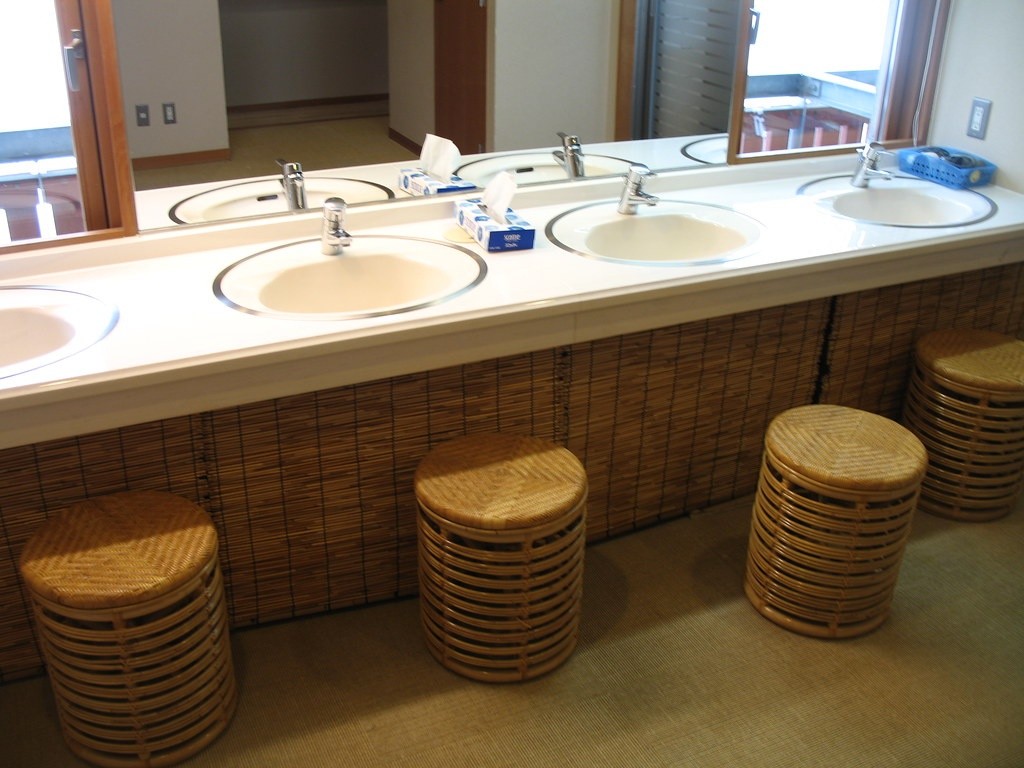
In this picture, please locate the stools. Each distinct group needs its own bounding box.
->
[745,405,927,638]
[413,432,591,683]
[904,326,1023,521]
[19,489,236,768]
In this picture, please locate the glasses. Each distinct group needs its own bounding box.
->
[921,147,977,169]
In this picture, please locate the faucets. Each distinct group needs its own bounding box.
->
[274,157,308,210]
[853,141,894,188]
[619,163,660,215]
[319,196,352,257]
[552,131,585,177]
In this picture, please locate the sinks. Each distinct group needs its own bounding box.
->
[257,253,450,313]
[203,190,347,222]
[829,188,974,225]
[0,305,76,368]
[479,162,614,183]
[584,213,748,259]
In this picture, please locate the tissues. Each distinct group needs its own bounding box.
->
[454,170,536,251]
[400,133,476,196]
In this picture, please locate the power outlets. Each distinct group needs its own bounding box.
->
[967,98,992,139]
[136,106,151,126]
[162,103,176,123]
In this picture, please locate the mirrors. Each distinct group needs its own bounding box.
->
[0,0,949,252]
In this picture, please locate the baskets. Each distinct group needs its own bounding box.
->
[898,144,998,191]
